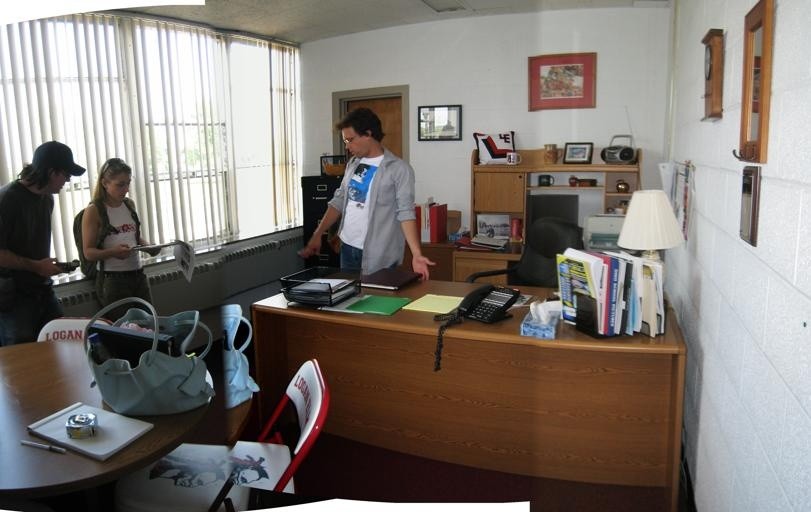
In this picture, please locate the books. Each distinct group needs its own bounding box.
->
[361,267,423,290]
[28,401,155,463]
[555,247,666,338]
[413,196,448,243]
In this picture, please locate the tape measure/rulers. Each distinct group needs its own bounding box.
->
[66,413,98,439]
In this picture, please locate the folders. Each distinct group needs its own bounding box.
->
[86,323,177,370]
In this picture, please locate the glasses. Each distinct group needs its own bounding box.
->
[50,166,72,182]
[102,158,125,175]
[342,128,370,145]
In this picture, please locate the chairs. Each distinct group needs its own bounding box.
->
[226,354,333,511]
[462,216,584,287]
[34,315,113,345]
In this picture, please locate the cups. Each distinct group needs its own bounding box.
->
[511,217,523,239]
[507,152,522,166]
[538,174,555,186]
[544,143,563,165]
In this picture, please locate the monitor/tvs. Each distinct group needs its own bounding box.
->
[527,193,579,224]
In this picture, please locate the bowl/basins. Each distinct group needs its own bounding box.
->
[615,208,624,214]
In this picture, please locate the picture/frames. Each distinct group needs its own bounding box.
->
[562,141,593,164]
[737,166,764,249]
[525,50,599,115]
[474,212,513,237]
[416,103,465,141]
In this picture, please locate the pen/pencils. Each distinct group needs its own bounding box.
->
[20,438,67,454]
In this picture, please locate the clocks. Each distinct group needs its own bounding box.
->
[699,26,728,124]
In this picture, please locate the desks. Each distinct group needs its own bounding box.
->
[1,337,214,511]
[219,388,254,440]
[247,285,691,511]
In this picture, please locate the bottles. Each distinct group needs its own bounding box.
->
[569,175,577,187]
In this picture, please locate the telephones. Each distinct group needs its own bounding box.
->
[457,284,520,325]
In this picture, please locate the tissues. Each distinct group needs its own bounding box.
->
[520,298,563,342]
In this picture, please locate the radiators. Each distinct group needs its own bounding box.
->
[50,257,217,331]
[218,233,304,301]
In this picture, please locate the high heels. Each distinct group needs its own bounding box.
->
[78,295,217,418]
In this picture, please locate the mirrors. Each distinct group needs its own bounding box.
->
[734,0,776,166]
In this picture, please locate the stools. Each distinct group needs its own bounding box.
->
[116,440,235,512]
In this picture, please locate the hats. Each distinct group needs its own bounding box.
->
[33,140,87,177]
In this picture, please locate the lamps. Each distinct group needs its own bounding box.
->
[615,188,687,289]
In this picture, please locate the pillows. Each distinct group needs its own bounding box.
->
[471,129,517,167]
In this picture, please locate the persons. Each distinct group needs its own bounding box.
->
[77,157,161,321]
[486,225,495,238]
[0,140,87,347]
[296,106,437,283]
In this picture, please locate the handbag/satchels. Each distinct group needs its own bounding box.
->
[215,302,263,411]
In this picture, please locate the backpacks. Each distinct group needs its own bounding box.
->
[72,199,141,279]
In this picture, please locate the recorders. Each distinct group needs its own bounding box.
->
[601,135,636,164]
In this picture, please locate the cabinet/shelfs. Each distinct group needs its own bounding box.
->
[451,243,523,283]
[398,208,461,283]
[466,147,644,243]
[298,173,344,269]
